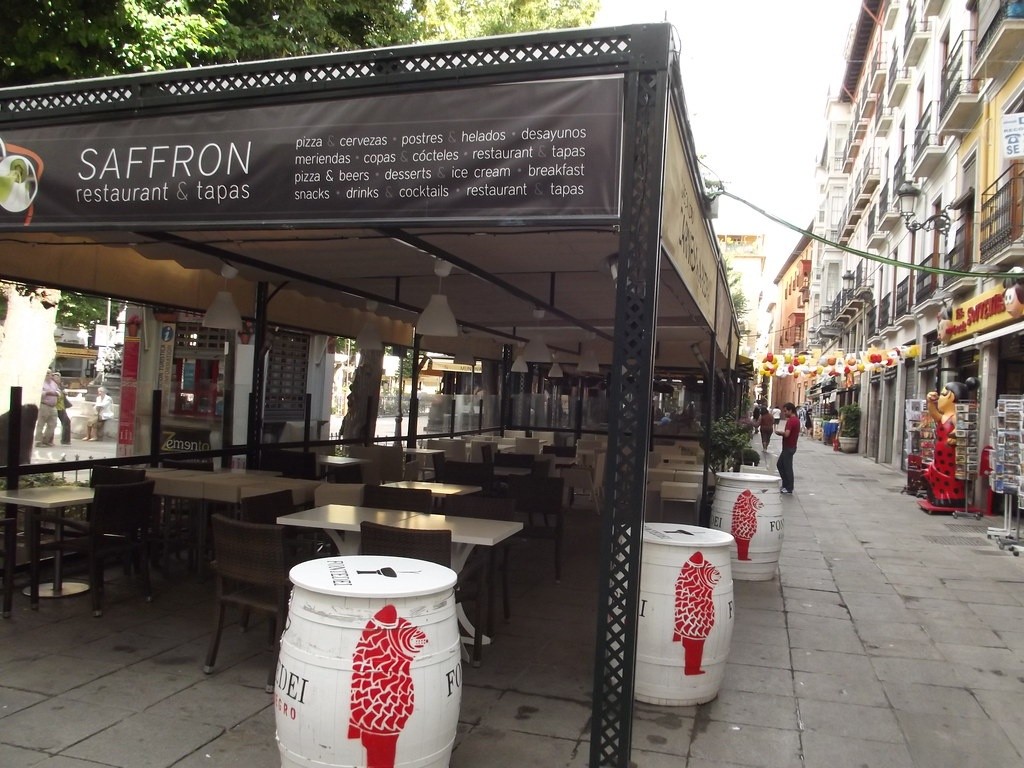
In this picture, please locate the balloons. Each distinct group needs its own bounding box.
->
[757,344,922,380]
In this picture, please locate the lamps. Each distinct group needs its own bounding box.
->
[842,271,875,304]
[896,180,952,239]
[520,307,551,363]
[454,333,474,365]
[576,330,599,372]
[415,258,460,339]
[201,290,243,332]
[822,307,843,332]
[547,353,563,378]
[509,341,528,373]
[355,310,384,352]
[809,327,830,347]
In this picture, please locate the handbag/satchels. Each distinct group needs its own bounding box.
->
[64,396,72,408]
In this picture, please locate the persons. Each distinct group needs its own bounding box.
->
[34,368,62,447]
[935,306,953,343]
[754,407,774,453]
[753,400,765,433]
[774,402,802,494]
[81,387,113,442]
[927,377,982,507]
[796,404,814,437]
[1002,276,1024,318]
[52,373,72,446]
[770,405,782,429]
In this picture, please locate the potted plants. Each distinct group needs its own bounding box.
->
[839,402,861,453]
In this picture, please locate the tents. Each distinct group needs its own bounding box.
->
[0,23,752,768]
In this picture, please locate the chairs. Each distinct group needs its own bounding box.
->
[0,429,726,698]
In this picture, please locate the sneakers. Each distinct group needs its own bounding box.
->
[781,487,788,493]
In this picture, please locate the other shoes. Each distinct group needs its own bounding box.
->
[35,442,54,447]
[82,436,103,441]
[61,443,73,447]
